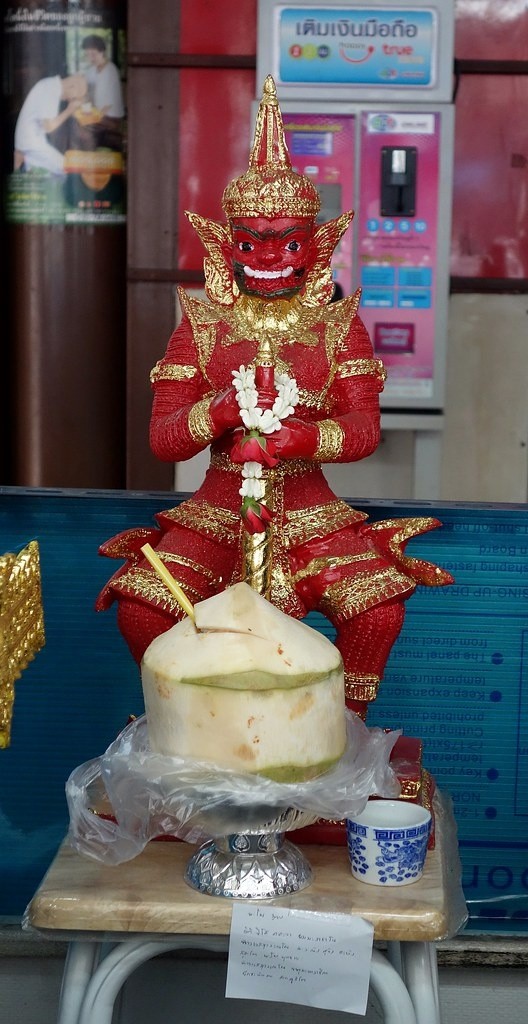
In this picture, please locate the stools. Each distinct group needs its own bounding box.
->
[27,786,449,1024]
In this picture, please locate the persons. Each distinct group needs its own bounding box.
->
[13,34,126,177]
[93,73,457,730]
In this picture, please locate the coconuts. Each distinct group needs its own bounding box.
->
[141,583,346,780]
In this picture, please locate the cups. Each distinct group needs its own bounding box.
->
[347,799,432,888]
